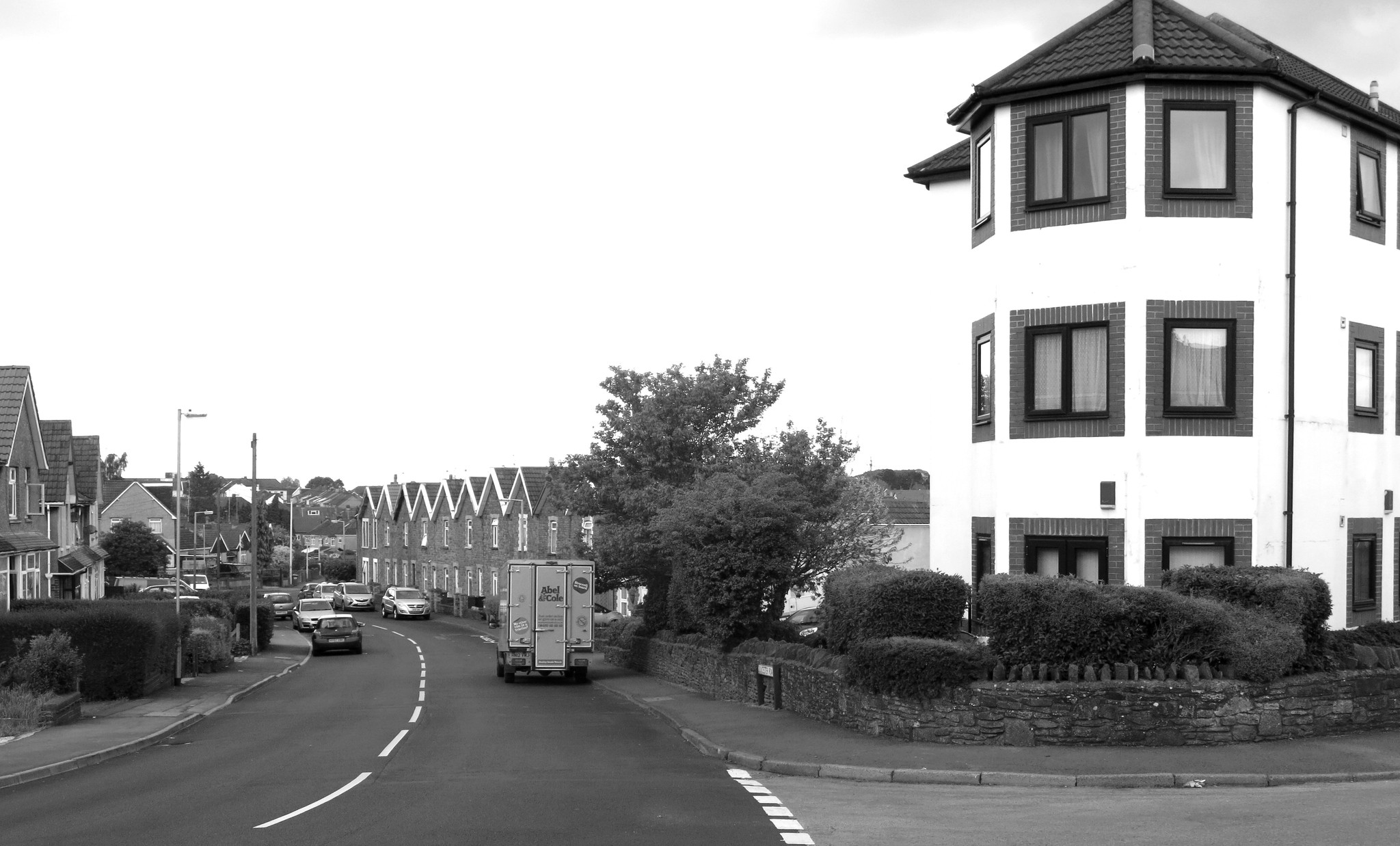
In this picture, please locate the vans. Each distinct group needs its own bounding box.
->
[183,574,212,590]
[115,577,197,591]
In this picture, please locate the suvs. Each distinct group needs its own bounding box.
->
[381,586,430,620]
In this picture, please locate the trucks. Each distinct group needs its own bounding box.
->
[488,559,596,683]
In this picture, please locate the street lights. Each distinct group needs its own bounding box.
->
[499,498,524,559]
[193,511,213,589]
[331,519,345,560]
[172,409,207,683]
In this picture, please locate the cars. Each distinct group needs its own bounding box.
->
[292,598,335,632]
[297,583,319,600]
[312,584,338,602]
[310,614,365,656]
[139,584,187,594]
[594,603,623,625]
[332,582,375,612]
[262,593,295,620]
